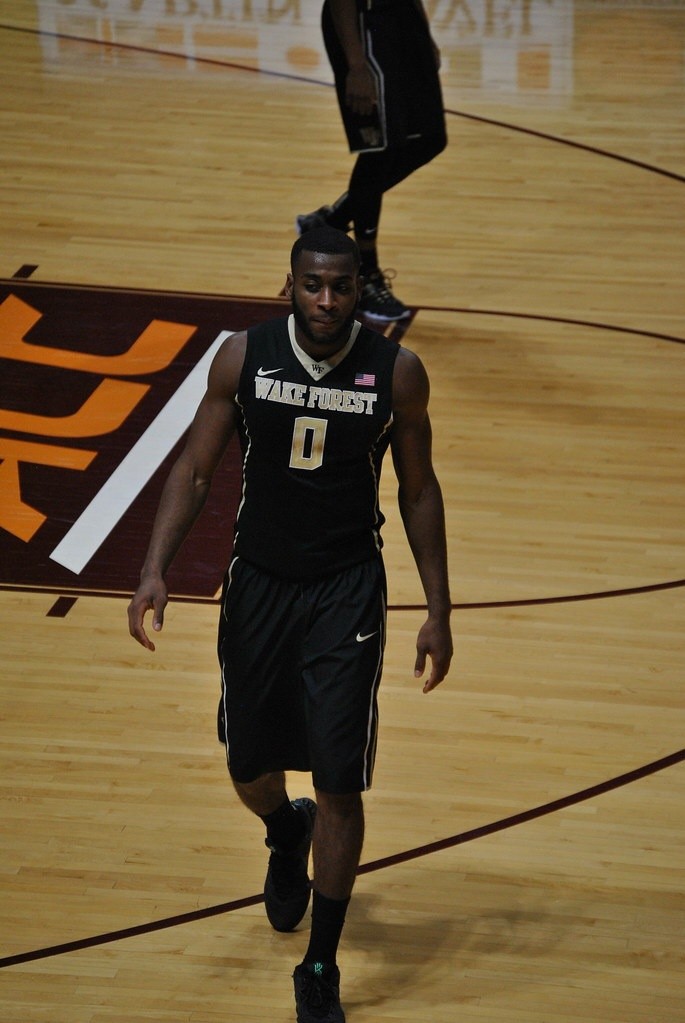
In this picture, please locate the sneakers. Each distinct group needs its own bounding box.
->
[358,268,411,321]
[296,203,351,237]
[291,963,345,1023]
[264,797,317,932]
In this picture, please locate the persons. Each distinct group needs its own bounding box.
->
[297,0,448,321]
[128,228,453,1023]
